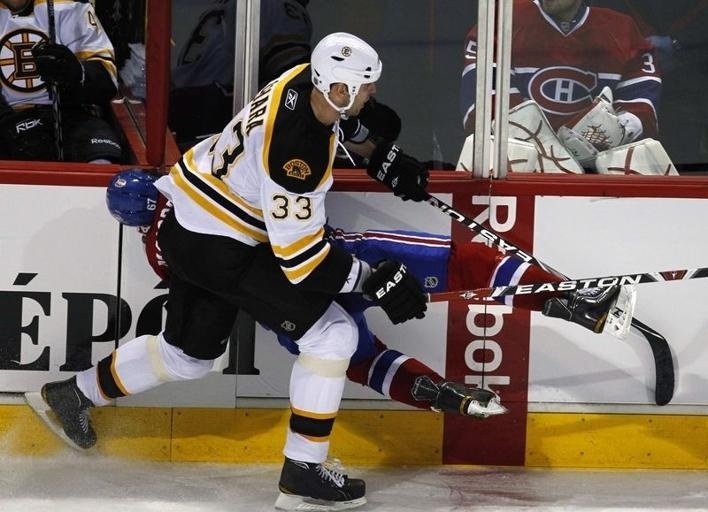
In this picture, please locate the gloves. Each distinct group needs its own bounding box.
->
[32,43,83,85]
[362,257,428,323]
[367,142,429,201]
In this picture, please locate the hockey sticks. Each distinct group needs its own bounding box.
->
[422,189,675,406]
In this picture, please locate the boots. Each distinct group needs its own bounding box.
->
[41,376,97,449]
[279,457,365,500]
[542,284,621,333]
[411,375,495,416]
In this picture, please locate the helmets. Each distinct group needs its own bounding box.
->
[106,169,158,225]
[311,32,383,97]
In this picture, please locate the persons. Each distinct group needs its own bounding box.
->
[453,0,680,176]
[39,32,430,503]
[0,1,131,165]
[162,0,317,149]
[104,163,622,418]
